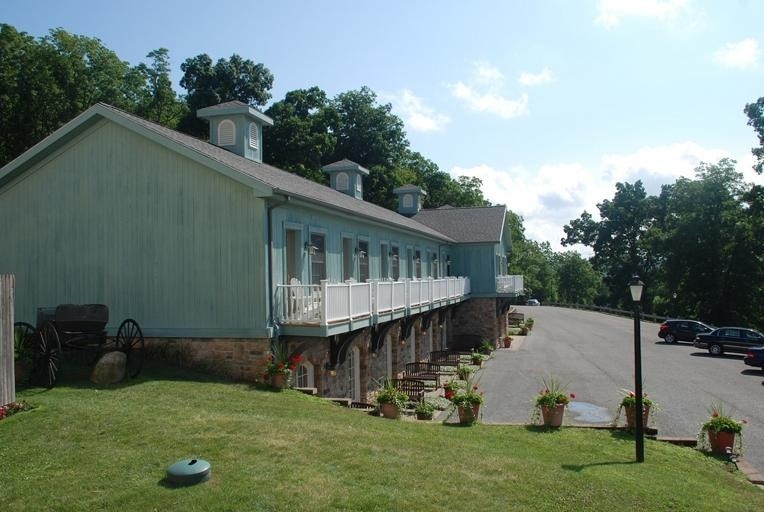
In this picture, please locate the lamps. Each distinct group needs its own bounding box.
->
[304,242,318,256]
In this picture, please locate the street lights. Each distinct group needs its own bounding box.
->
[627,273,646,464]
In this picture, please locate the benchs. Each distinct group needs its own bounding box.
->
[392,340,475,408]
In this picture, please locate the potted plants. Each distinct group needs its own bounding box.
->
[470,337,496,365]
[503,336,513,348]
[265,341,304,380]
[371,376,434,420]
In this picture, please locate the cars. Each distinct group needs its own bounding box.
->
[657,319,714,345]
[526,299,541,306]
[693,326,764,357]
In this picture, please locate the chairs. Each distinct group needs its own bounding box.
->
[290,277,314,320]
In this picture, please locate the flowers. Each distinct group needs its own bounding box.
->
[696,399,748,451]
[616,376,657,418]
[444,370,488,420]
[530,371,575,420]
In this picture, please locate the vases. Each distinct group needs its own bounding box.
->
[625,407,649,426]
[541,404,564,427]
[457,402,479,424]
[708,430,735,453]
[270,371,289,389]
